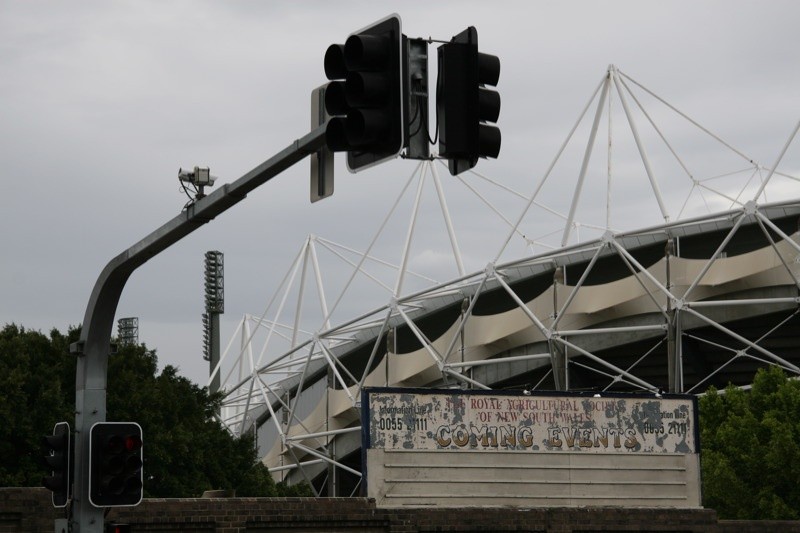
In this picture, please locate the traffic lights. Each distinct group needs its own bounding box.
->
[90,422,144,508]
[323,13,403,174]
[438,26,501,175]
[40,422,69,508]
